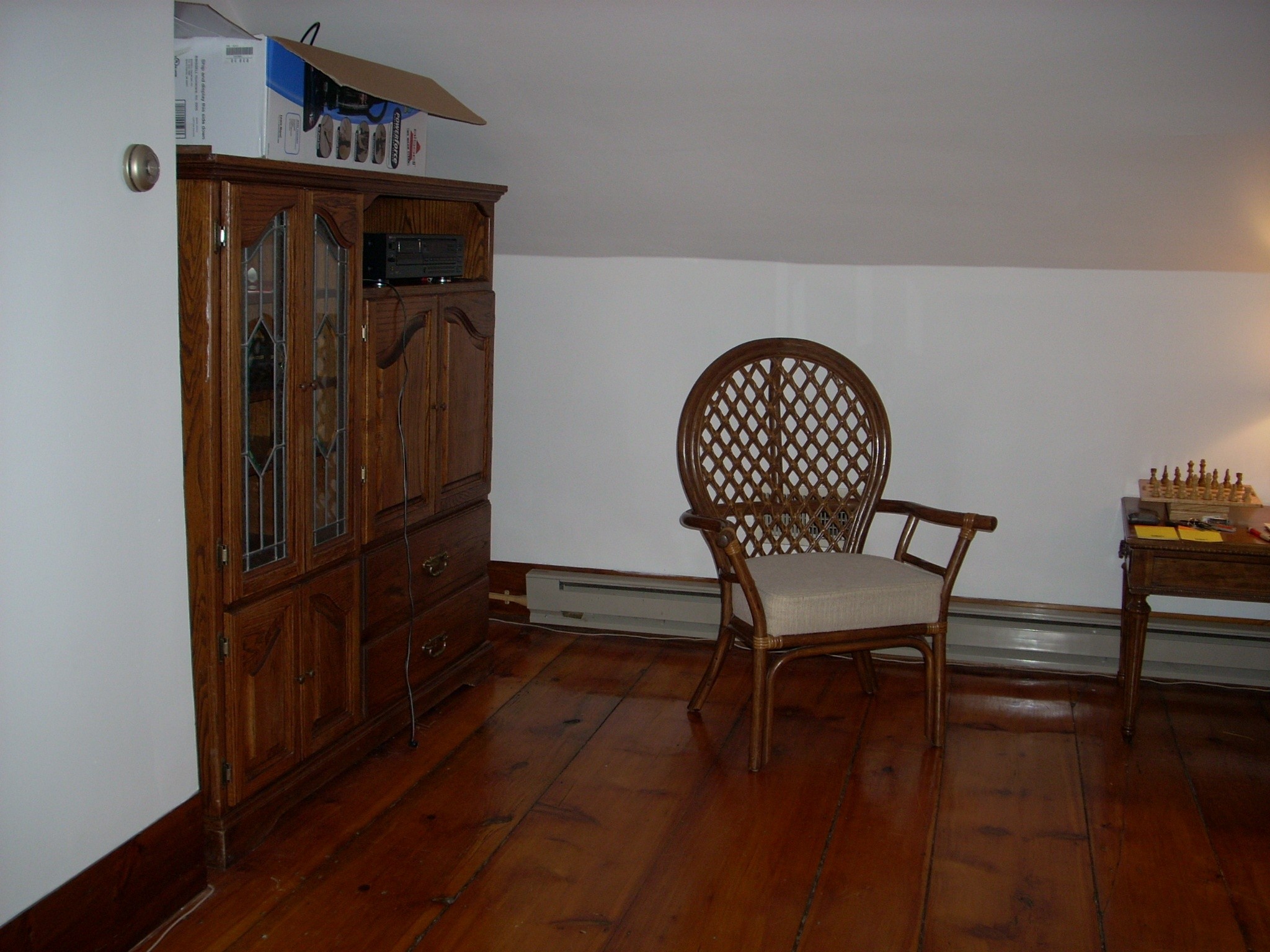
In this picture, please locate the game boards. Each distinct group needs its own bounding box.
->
[1138,479,1263,506]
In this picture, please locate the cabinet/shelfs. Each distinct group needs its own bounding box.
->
[177,153,509,873]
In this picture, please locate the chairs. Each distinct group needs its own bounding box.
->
[675,337,999,774]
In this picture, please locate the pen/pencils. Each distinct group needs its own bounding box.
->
[1246,527,1270,542]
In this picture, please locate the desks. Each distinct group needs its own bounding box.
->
[1114,497,1270,746]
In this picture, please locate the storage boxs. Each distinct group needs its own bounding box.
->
[173,1,489,179]
